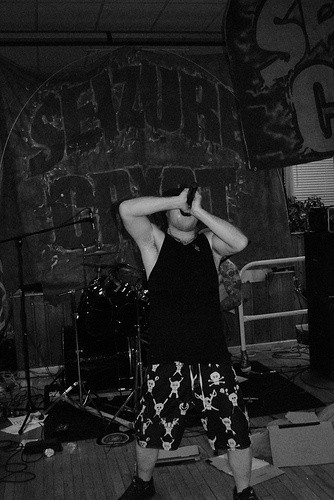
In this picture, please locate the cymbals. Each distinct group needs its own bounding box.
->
[77,250,119,257]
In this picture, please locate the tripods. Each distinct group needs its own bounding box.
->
[0,217,89,436]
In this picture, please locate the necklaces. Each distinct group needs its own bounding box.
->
[168,226,196,245]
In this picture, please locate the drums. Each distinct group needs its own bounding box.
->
[62,326,132,394]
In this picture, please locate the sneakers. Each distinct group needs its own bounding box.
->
[232,486,260,500]
[117,476,156,499]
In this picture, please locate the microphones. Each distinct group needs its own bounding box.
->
[180,182,198,216]
[89,211,95,230]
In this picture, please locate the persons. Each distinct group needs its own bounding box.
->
[112,181,261,500]
[188,224,243,312]
